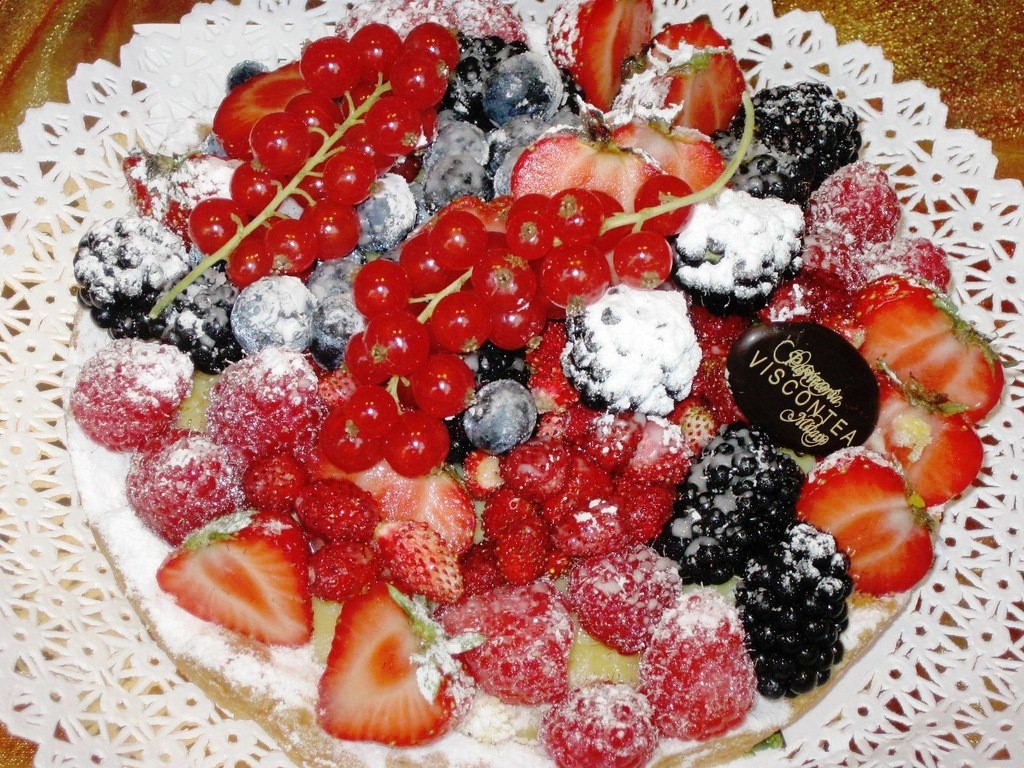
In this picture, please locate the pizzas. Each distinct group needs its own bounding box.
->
[60,0,1005,768]
